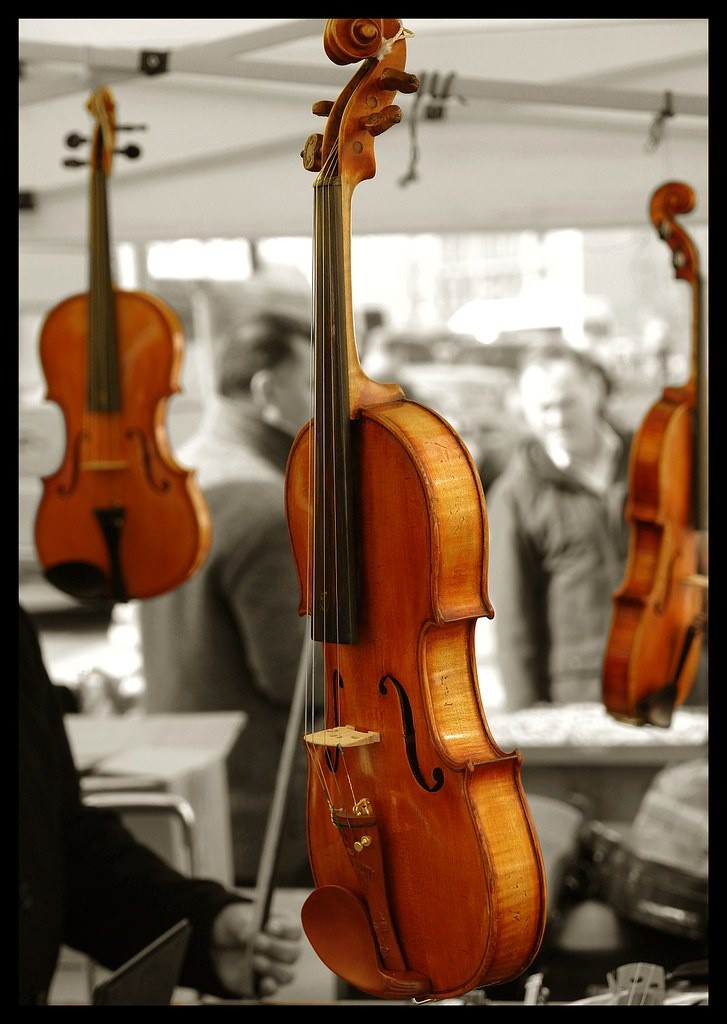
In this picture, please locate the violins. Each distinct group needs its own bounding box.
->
[34,83,214,605]
[284,20,549,1005]
[600,182,708,729]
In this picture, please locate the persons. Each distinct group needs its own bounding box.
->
[15,603,305,1007]
[146,311,642,890]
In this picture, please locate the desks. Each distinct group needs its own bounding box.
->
[64,709,249,889]
[497,704,709,826]
